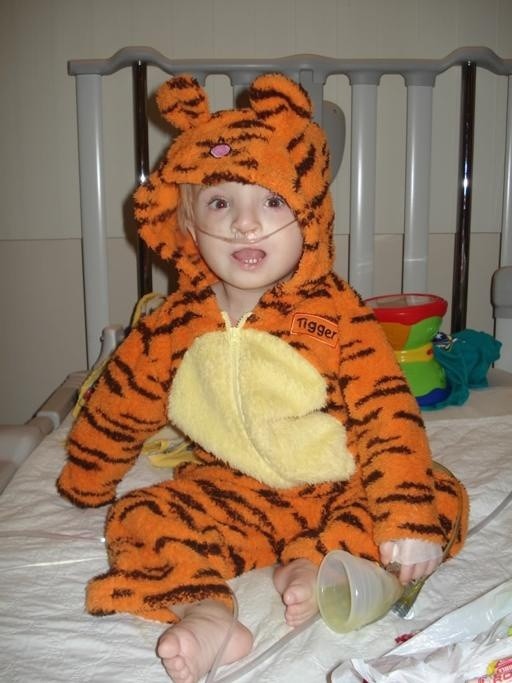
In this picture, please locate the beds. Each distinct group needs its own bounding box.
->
[1,45,512,683]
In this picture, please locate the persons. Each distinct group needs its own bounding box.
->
[54,71,468,683]
